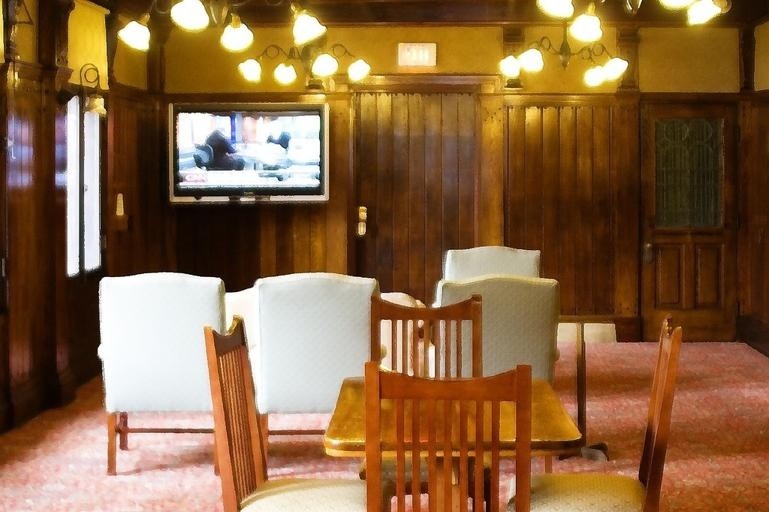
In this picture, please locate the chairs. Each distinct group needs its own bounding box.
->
[357,292,497,512]
[367,357,533,511]
[199,311,368,512]
[504,314,683,511]
[98,240,565,476]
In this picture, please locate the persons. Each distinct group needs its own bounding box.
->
[267,126,292,170]
[195,121,245,171]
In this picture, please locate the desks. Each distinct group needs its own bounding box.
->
[322,370,586,509]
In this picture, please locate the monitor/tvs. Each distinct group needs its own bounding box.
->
[167,103,329,205]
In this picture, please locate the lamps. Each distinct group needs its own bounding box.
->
[495,0,731,92]
[59,62,107,118]
[117,0,372,86]
